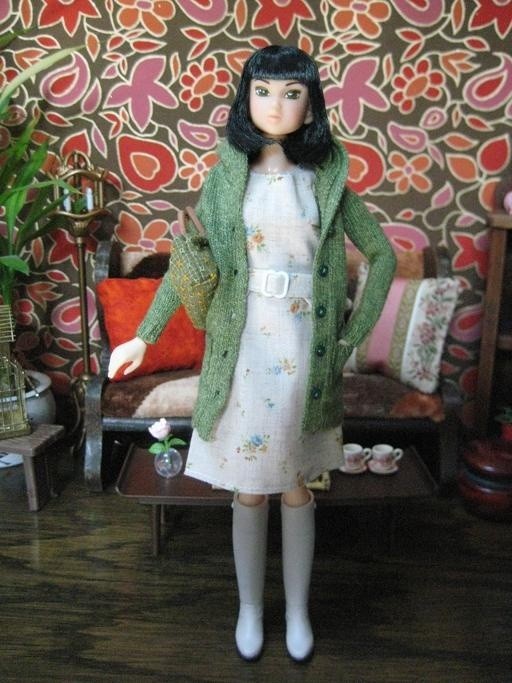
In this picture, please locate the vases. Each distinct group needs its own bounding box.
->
[155,447,182,477]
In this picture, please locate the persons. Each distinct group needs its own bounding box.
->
[108,45,399,661]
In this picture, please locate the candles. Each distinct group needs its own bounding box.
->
[85,187,94,210]
[62,187,70,211]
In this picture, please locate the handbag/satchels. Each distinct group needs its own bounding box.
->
[169,209,220,330]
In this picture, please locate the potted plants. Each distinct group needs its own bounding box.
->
[496,406,512,440]
[0,24,99,440]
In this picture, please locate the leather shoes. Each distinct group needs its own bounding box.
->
[247,268,315,301]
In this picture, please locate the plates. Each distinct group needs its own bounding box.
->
[368,459,398,474]
[338,465,366,473]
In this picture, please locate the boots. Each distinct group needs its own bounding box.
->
[232,492,268,660]
[281,493,315,662]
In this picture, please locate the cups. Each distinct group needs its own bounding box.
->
[341,443,371,469]
[371,443,403,468]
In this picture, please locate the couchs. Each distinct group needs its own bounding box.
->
[83,239,462,491]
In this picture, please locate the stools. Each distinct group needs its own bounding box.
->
[0,423,65,511]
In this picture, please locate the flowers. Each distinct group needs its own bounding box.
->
[147,417,187,468]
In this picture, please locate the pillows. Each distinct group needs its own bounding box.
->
[93,277,204,380]
[346,260,458,393]
